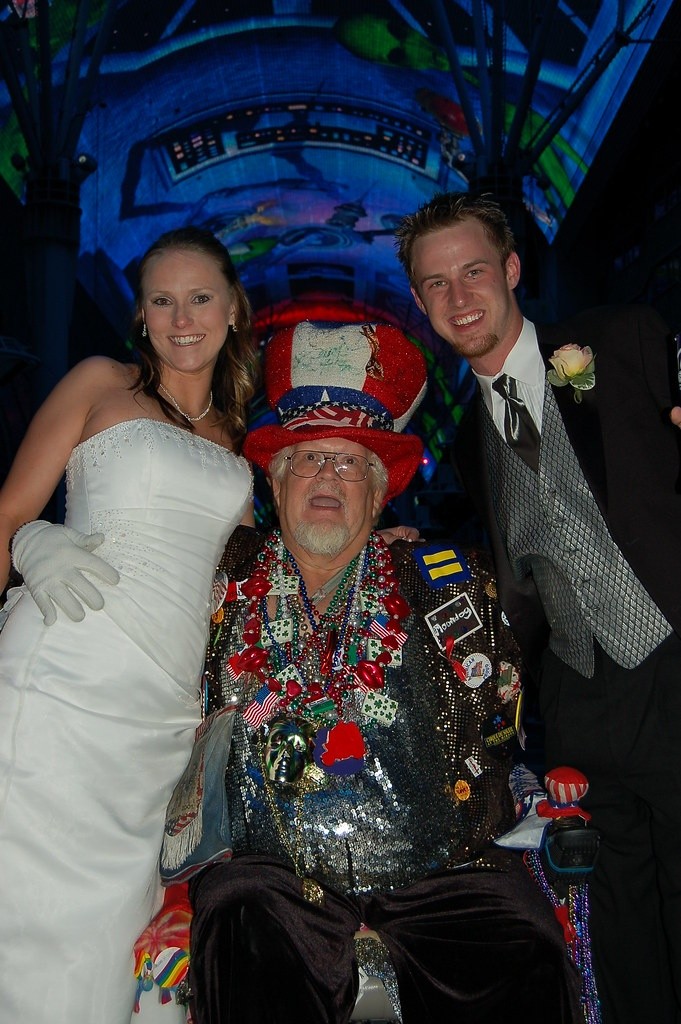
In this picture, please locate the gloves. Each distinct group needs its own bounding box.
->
[10,519,121,626]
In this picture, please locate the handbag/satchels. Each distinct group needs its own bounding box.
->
[158,705,234,887]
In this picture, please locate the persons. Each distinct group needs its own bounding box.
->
[0,227,429,1024]
[188,320,582,1024]
[395,191,681,1024]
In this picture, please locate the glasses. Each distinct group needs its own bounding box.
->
[283,450,375,482]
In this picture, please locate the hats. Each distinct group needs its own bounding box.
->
[241,319,428,508]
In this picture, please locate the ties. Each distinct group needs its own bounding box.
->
[492,373,541,475]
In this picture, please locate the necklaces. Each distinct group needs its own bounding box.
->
[235,524,410,906]
[159,384,213,421]
